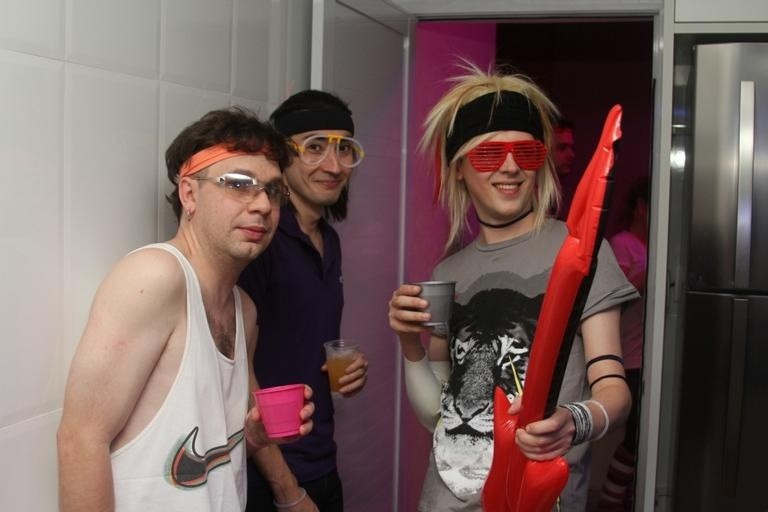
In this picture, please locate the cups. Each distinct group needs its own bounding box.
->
[252,384,306,438]
[325,339,359,395]
[411,280,457,326]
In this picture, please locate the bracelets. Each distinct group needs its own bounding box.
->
[560,395,608,445]
[274,487,307,509]
[588,374,630,391]
[585,354,624,374]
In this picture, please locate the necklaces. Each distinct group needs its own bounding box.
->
[478,206,532,229]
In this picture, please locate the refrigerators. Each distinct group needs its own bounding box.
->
[667,42,763,510]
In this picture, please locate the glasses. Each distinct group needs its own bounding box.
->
[193,172,291,206]
[285,134,366,168]
[463,140,549,172]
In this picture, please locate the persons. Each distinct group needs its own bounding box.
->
[600,180,650,512]
[56,107,315,512]
[551,130,581,221]
[388,53,640,512]
[237,90,370,512]
